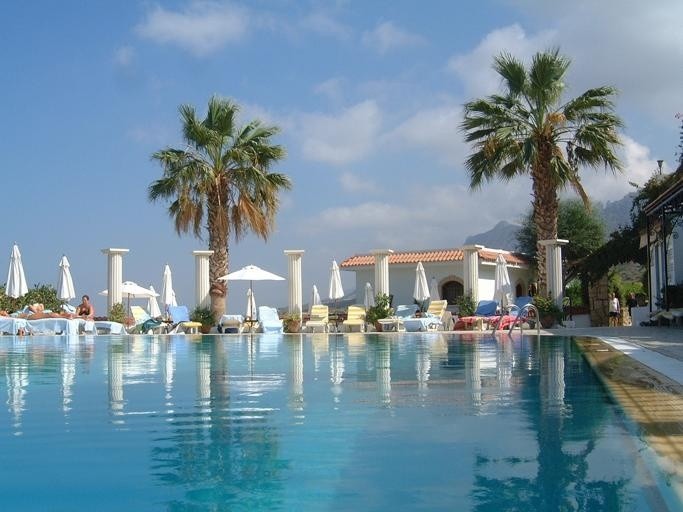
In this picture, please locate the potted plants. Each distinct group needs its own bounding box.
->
[283,313,303,332]
[532,297,557,329]
[193,305,218,332]
[368,294,393,331]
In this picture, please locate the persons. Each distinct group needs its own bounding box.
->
[626,292,637,325]
[413,309,427,318]
[607,293,621,328]
[0,295,95,337]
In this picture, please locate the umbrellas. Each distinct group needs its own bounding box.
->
[308,283,322,316]
[55,254,76,311]
[413,260,430,316]
[492,253,512,318]
[327,256,345,330]
[98,280,160,331]
[430,276,439,301]
[363,282,374,313]
[216,264,287,319]
[161,262,172,321]
[146,283,161,320]
[5,240,29,312]
[161,289,178,312]
[246,288,256,321]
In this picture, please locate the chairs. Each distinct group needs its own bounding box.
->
[129,305,201,335]
[1,303,123,336]
[306,303,365,332]
[257,307,284,332]
[218,314,244,333]
[379,296,536,331]
